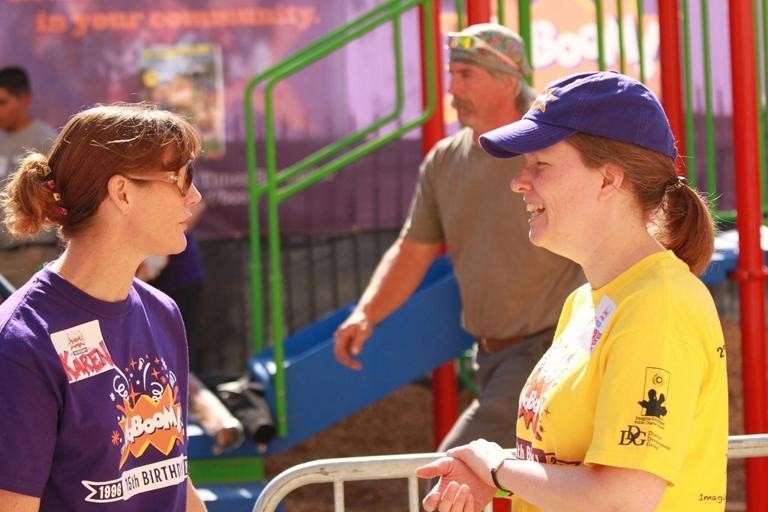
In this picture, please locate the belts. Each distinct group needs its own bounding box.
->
[475,327,548,355]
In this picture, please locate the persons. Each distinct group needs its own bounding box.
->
[0,103,209,512]
[415,70,730,512]
[333,22,590,453]
[0,65,66,291]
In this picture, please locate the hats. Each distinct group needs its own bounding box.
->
[479,70,678,160]
[445,23,530,78]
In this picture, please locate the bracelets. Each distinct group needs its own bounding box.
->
[491,458,515,497]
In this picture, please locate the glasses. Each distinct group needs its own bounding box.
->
[448,35,483,54]
[125,161,194,194]
